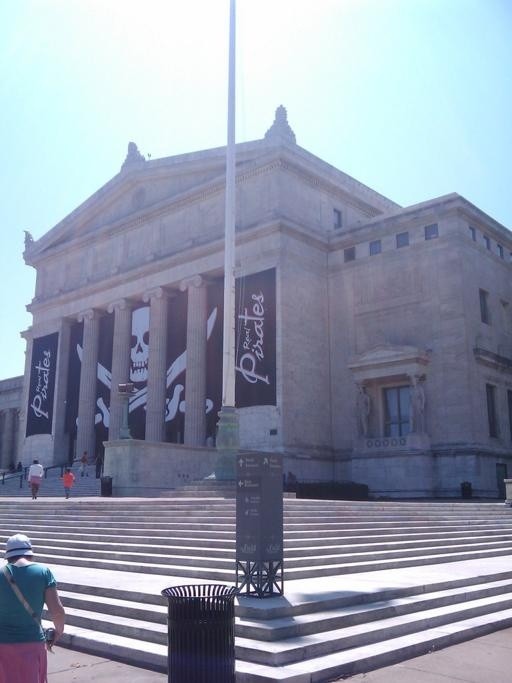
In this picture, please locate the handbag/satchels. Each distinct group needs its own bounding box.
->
[30,476,40,484]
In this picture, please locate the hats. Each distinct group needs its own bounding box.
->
[6,535,33,561]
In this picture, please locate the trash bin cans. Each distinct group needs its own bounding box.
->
[100,476,112,496]
[161,584,240,683]
[461,482,472,499]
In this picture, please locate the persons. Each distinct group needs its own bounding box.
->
[58,467,77,500]
[78,450,90,476]
[28,458,45,499]
[93,449,102,479]
[1,529,68,682]
